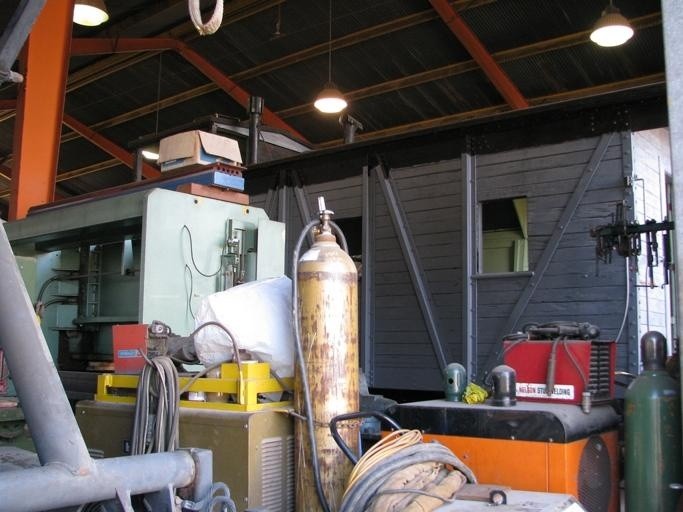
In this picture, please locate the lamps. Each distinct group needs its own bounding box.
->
[588,1,635,46]
[70,1,113,28]
[312,1,346,117]
[141,33,174,162]
[338,109,362,144]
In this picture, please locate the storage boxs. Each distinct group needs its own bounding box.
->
[156,128,242,175]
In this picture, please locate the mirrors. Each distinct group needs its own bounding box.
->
[472,194,530,275]
[325,212,365,279]
